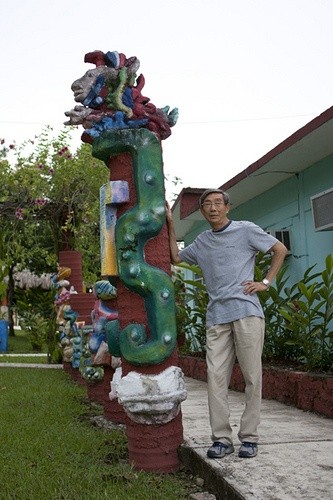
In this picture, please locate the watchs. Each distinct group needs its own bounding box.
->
[262,278,270,287]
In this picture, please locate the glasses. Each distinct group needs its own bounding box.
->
[202,202,223,207]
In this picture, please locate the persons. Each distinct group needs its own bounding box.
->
[165,189,288,458]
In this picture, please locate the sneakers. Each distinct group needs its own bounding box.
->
[239,442,258,458]
[207,443,235,458]
